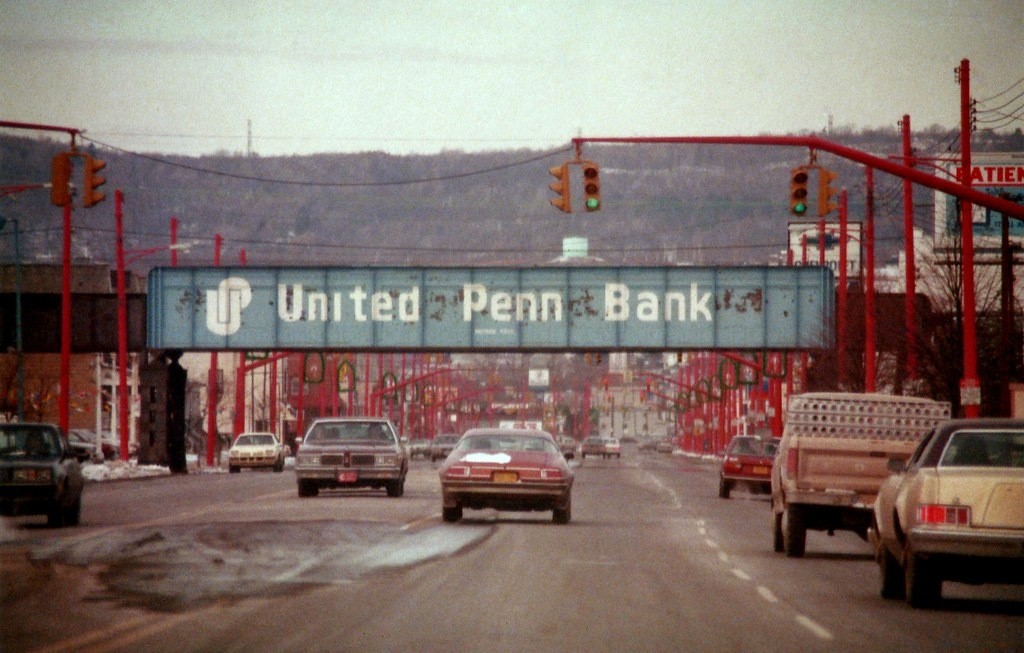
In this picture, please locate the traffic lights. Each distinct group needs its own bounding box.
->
[819,168,837,215]
[549,165,570,214]
[84,156,107,209]
[582,164,601,211]
[790,169,808,216]
[595,352,600,366]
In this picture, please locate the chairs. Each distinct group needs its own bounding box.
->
[952,436,990,467]
[475,439,491,449]
[327,427,340,438]
[23,431,44,453]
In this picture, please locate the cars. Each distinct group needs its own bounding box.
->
[430,434,461,461]
[68,428,132,461]
[604,439,621,458]
[408,439,431,457]
[438,428,576,523]
[228,432,285,473]
[0,422,83,526]
[581,437,607,459]
[871,416,1024,609]
[656,442,673,453]
[719,435,781,498]
[294,418,410,497]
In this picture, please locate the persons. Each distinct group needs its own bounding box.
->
[314,424,327,440]
[367,423,385,439]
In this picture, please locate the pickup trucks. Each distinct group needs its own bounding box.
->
[765,393,922,558]
